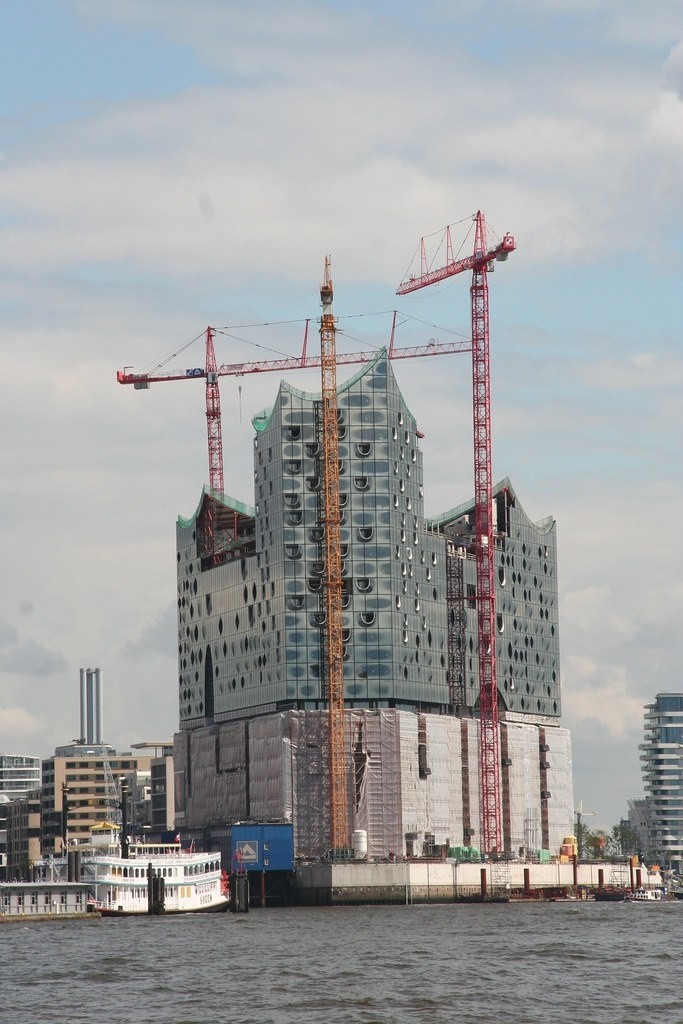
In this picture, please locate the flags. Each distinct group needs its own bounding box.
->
[174,833,180,843]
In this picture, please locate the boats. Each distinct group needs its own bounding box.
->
[670,875,683,899]
[626,886,662,901]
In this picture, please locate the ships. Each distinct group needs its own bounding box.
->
[30,777,230,918]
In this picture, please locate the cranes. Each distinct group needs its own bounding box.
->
[114,309,478,568]
[318,253,348,858]
[396,209,516,858]
